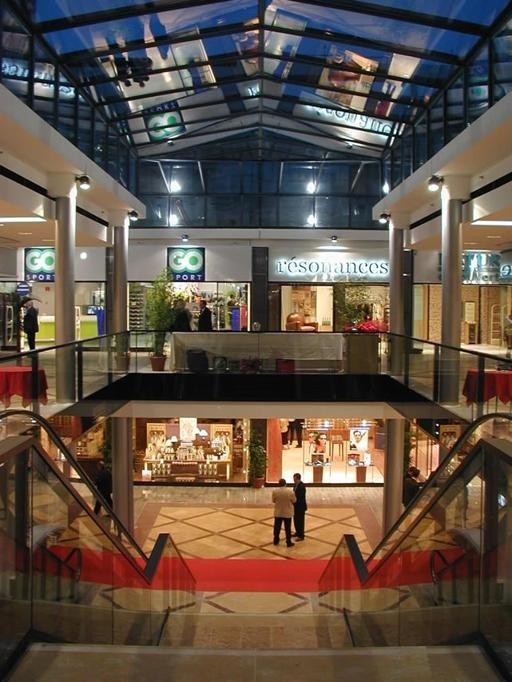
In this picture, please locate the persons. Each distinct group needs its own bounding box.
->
[173,299,192,331]
[105,13,172,88]
[271,479,297,547]
[352,431,365,448]
[24,302,39,350]
[292,473,307,541]
[403,469,420,506]
[404,466,416,479]
[309,433,314,443]
[280,418,289,448]
[314,433,326,453]
[93,460,113,516]
[287,419,295,445]
[198,300,212,331]
[295,419,305,448]
[171,300,179,313]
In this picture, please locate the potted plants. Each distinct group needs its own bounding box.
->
[248,429,268,489]
[113,330,132,372]
[146,267,174,371]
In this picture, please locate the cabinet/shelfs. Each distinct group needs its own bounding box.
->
[306,418,377,440]
[143,433,232,480]
[182,291,217,328]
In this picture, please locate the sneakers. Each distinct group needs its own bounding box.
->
[286,533,303,547]
[274,541,279,544]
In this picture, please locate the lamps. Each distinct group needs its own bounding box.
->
[428,176,443,191]
[128,211,138,222]
[76,175,90,189]
[182,235,189,242]
[379,214,391,223]
[332,236,337,242]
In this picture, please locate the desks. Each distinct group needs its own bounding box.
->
[463,368,512,425]
[0,366,49,409]
